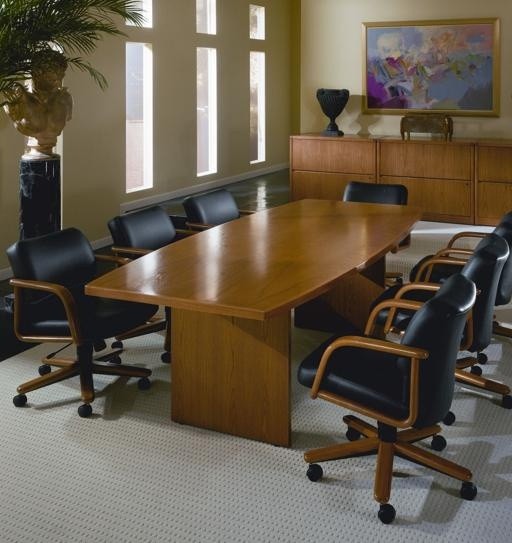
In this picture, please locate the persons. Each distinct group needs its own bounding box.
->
[3,49,74,148]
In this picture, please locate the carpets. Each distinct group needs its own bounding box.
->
[0,220,510,542]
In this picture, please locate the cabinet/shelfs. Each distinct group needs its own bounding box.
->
[289,136,512,229]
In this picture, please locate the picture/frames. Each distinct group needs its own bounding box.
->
[360,16,502,118]
